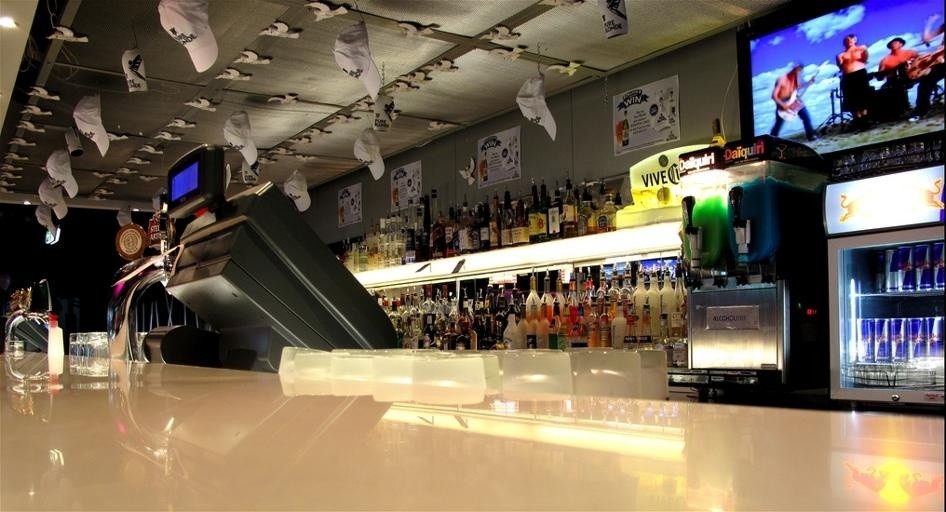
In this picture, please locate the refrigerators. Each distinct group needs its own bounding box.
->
[821,160,946,407]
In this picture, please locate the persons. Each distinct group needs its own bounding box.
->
[878,38,920,95]
[835,34,869,132]
[908,13,945,123]
[770,58,818,141]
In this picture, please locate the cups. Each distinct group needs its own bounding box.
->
[68,331,110,364]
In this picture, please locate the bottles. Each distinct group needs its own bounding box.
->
[345,169,622,274]
[381,259,687,349]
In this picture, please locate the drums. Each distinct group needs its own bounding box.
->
[886,60,915,89]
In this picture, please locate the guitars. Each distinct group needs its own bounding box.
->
[908,44,946,79]
[778,60,827,121]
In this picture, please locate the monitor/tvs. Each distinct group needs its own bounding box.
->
[163,179,397,377]
